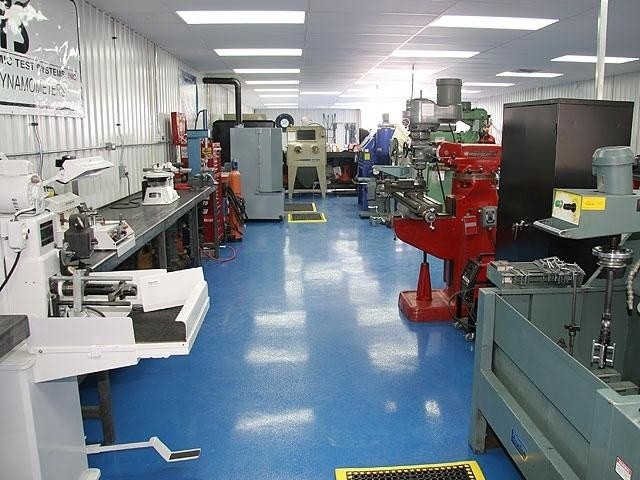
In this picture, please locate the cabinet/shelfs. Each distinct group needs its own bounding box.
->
[495,98,634,285]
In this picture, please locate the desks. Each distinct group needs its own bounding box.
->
[68,184,218,272]
